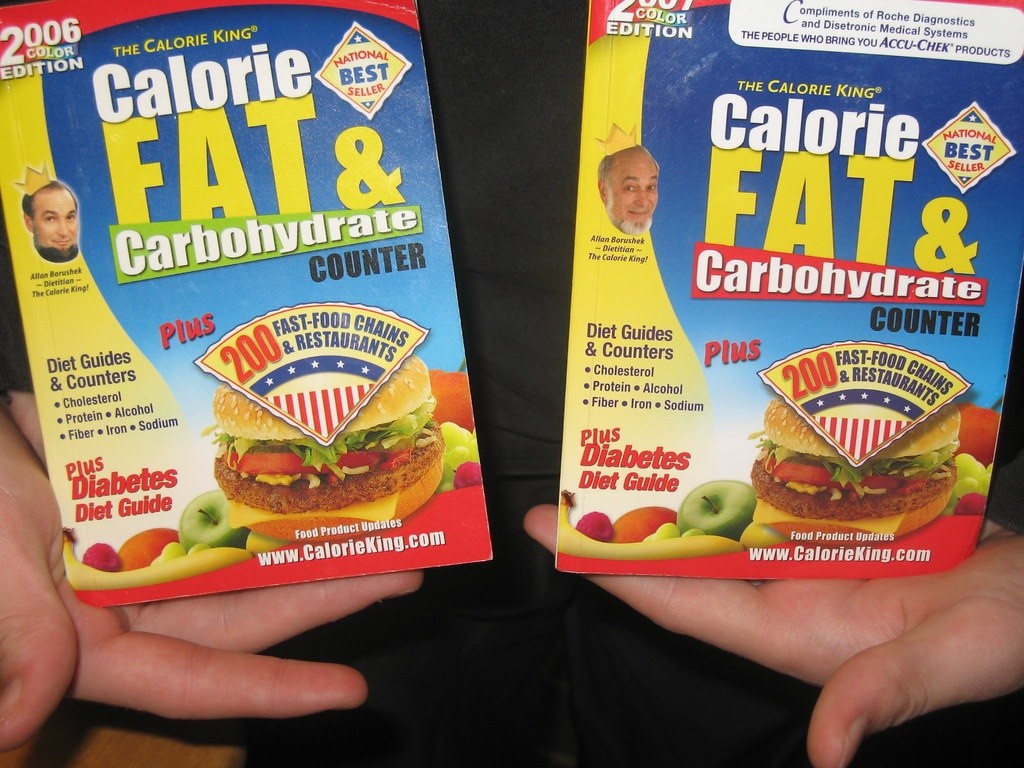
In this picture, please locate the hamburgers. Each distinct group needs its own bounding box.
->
[205,353,444,542]
[747,395,961,541]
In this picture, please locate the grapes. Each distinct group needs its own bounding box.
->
[150,542,211,567]
[433,421,483,495]
[941,453,994,518]
[641,523,706,543]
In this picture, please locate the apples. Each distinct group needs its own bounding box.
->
[607,479,791,550]
[117,490,294,572]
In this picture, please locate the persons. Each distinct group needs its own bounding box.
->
[22,180,82,263]
[598,145,661,235]
[0,1,1024,768]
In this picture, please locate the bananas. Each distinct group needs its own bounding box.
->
[63,527,255,590]
[558,490,745,561]
